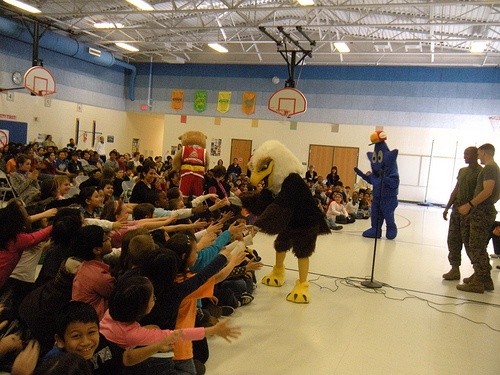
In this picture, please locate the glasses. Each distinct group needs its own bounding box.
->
[148,294,156,305]
[103,232,109,243]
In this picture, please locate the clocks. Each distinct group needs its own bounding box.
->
[12,71,23,85]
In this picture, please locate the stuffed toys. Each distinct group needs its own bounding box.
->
[247,140,332,303]
[173,131,210,204]
[354,130,400,239]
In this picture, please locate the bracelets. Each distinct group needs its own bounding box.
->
[469,202,473,207]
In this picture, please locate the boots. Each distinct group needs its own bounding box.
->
[443,265,460,280]
[463,273,476,283]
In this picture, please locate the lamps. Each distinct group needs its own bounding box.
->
[0,0,500,52]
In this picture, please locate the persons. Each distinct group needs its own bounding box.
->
[489,222,500,258]
[0,135,266,375]
[456,144,500,293]
[442,146,484,280]
[304,164,373,230]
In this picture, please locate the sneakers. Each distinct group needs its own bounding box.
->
[457,284,484,293]
[468,279,494,290]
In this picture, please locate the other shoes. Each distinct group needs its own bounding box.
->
[490,253,500,258]
[237,296,252,306]
[219,305,235,316]
[242,292,255,300]
[332,225,343,230]
[253,282,257,289]
